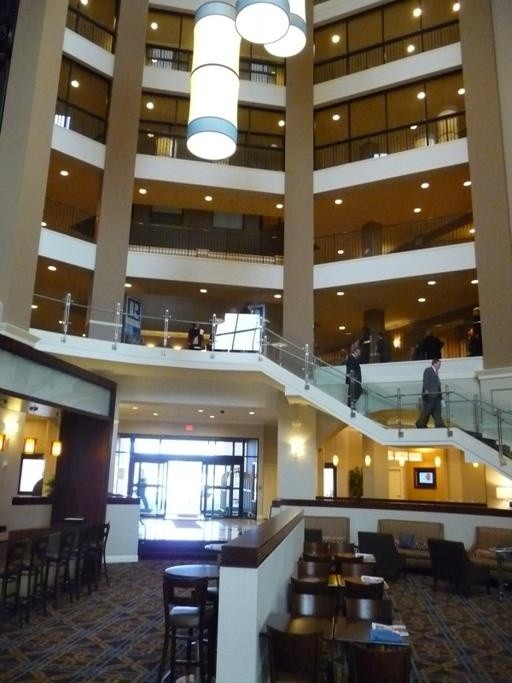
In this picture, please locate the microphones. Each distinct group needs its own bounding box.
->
[156,543,228,683]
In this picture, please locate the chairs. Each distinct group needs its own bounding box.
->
[266,516,410,683]
[0,522,111,634]
[358,515,511,599]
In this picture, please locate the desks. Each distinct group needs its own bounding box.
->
[259,614,335,682]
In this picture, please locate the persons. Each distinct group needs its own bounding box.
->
[346,347,363,411]
[375,332,391,362]
[423,330,444,358]
[187,324,196,346]
[471,307,482,356]
[412,341,424,359]
[358,327,372,363]
[415,356,447,428]
[136,479,150,511]
[193,327,204,349]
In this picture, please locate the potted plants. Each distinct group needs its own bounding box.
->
[414,468,436,489]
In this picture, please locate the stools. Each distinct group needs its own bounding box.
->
[156,543,228,683]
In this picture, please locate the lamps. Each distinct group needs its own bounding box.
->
[1,433,62,457]
[0,522,111,634]
[186,0,306,160]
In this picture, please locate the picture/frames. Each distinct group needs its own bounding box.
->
[414,468,436,489]
[259,614,335,682]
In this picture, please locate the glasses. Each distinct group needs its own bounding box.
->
[1,433,62,457]
[186,0,306,160]
